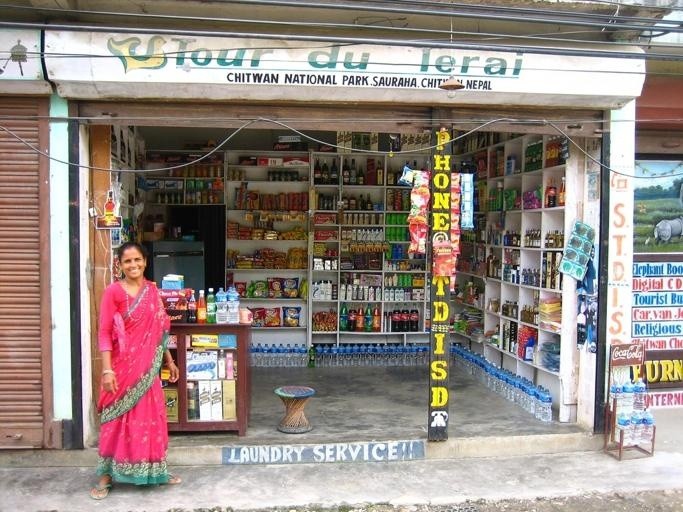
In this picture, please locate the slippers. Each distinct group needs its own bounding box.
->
[162,473,183,485]
[88,476,113,501]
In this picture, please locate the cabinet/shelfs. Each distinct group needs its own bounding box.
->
[311,152,459,347]
[160,323,254,437]
[458,133,586,423]
[225,150,312,350]
[145,149,225,205]
[89,125,145,429]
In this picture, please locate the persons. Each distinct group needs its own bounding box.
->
[90,242,181,500]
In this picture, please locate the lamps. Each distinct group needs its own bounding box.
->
[438,20,465,99]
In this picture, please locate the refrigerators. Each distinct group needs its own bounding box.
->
[149,239,205,296]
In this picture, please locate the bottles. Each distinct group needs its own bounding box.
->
[216,349,227,378]
[610,376,655,446]
[227,167,308,212]
[456,131,566,372]
[249,342,430,369]
[312,156,430,334]
[103,191,116,227]
[450,342,553,422]
[154,162,223,204]
[576,292,589,348]
[187,284,240,325]
[187,383,197,421]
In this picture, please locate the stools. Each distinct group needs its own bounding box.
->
[274,385,316,434]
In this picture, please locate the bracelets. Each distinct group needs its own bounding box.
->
[102,370,114,375]
[167,360,175,366]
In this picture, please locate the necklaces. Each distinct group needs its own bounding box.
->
[125,278,149,322]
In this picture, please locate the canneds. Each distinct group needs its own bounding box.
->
[278,192,309,210]
[228,167,246,181]
[343,214,383,225]
[358,286,363,299]
[191,192,201,204]
[268,171,299,181]
[353,286,358,299]
